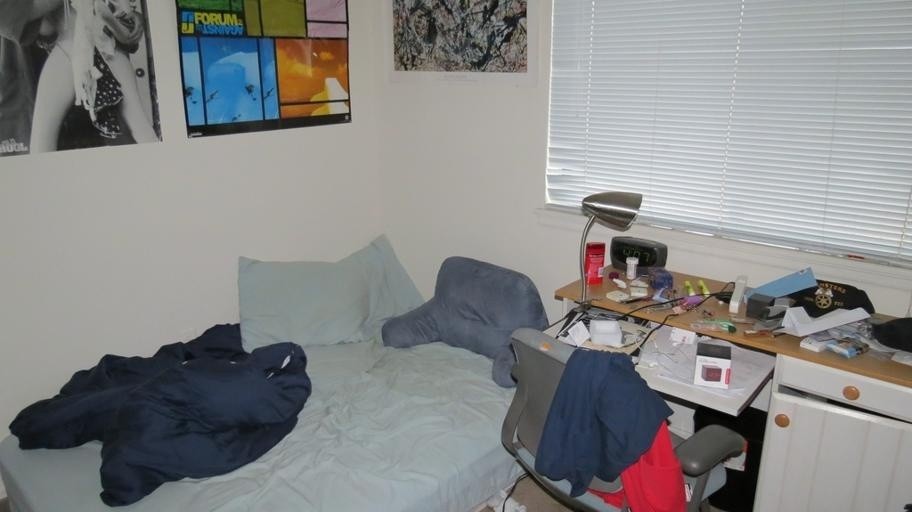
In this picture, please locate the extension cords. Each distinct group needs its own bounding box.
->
[728,275,748,314]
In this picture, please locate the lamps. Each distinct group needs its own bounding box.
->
[580,191,643,309]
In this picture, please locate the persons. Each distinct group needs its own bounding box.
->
[0,2,67,156]
[24,0,163,154]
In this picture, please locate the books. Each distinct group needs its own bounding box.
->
[657,336,777,398]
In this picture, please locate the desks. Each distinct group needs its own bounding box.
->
[556,264,912,511]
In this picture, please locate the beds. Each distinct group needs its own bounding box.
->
[0,347,528,510]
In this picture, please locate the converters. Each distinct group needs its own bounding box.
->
[745,293,776,319]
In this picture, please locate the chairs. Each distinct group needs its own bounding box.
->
[504,327,743,512]
